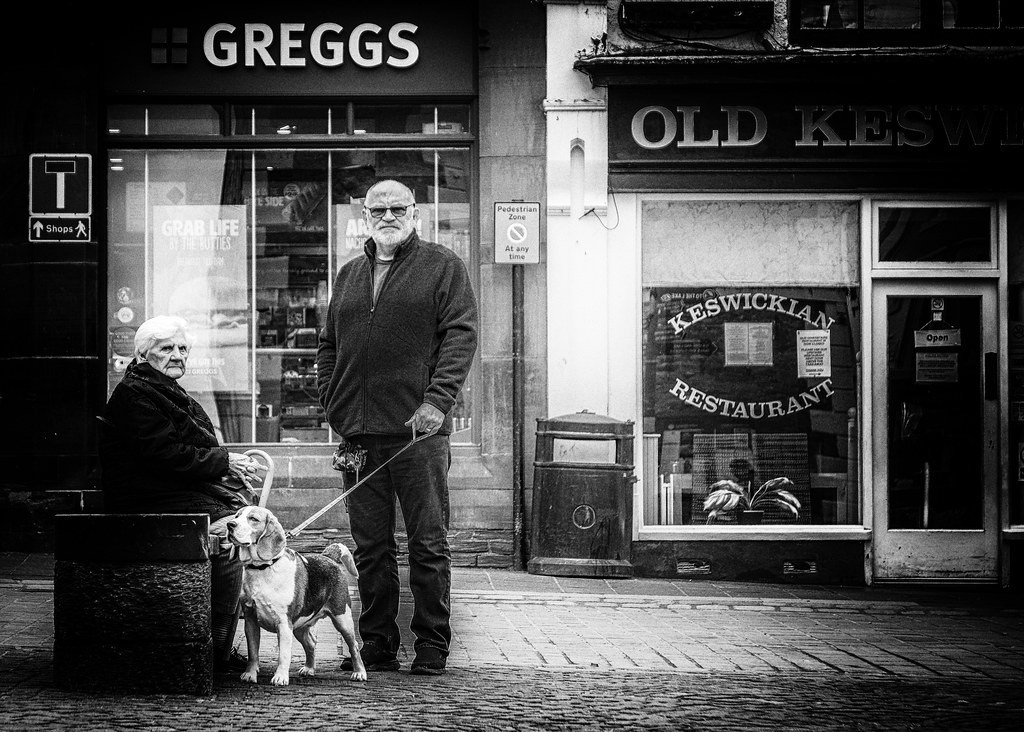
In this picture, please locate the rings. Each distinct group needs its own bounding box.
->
[425,428,431,431]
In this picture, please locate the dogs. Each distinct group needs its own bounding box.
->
[227,506,367,686]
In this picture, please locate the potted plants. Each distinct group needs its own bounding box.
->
[703,477,800,525]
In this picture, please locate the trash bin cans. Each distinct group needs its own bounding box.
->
[530,408,635,581]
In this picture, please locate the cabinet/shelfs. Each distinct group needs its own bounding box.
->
[253,284,326,431]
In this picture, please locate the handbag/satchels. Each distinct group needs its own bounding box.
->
[207,465,260,511]
[332,440,368,473]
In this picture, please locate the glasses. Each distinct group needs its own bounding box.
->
[364,203,414,218]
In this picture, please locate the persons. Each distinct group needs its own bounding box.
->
[93,311,269,678]
[317,178,477,676]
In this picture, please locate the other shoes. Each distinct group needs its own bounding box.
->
[229,647,247,674]
[409,648,446,675]
[340,644,400,671]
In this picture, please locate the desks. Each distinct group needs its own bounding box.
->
[660,471,846,526]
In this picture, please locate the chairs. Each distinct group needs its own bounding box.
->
[751,434,811,526]
[691,433,752,526]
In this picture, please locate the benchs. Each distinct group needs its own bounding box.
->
[46,512,218,697]
